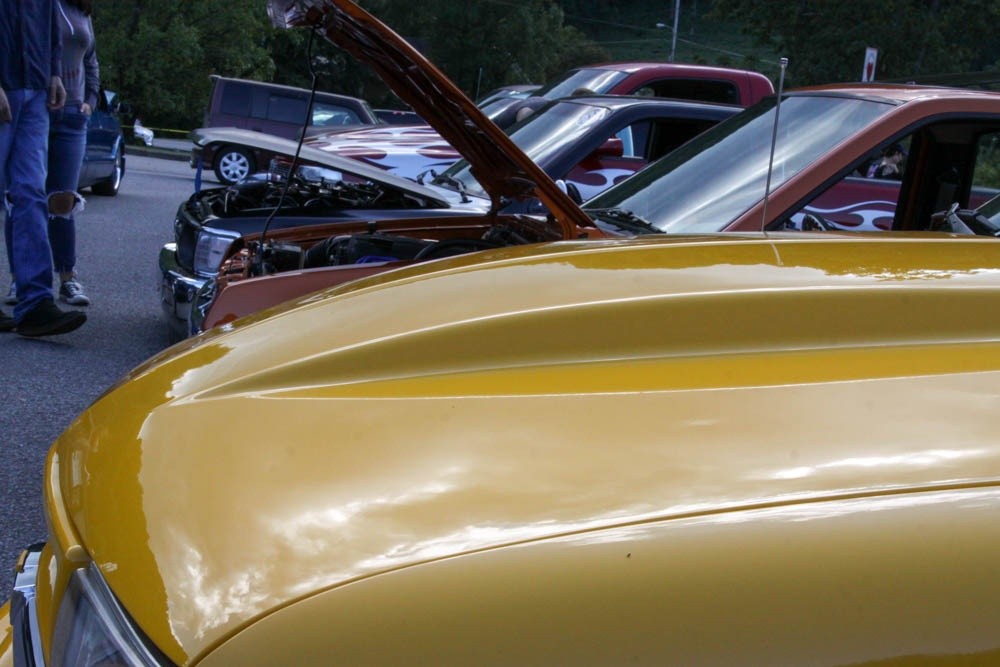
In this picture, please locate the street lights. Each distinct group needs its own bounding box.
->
[656,22,678,63]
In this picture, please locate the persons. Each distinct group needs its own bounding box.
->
[867,144,908,178]
[0,0,100,336]
[490,88,596,130]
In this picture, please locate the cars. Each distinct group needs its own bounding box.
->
[133,119,154,147]
[187,0,1000,337]
[75,81,128,196]
[156,58,1000,332]
[1,227,999,667]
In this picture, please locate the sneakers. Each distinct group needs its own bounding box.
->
[4,278,18,305]
[0,310,16,330]
[59,281,90,306]
[17,301,87,337]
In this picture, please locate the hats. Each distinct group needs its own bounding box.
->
[886,144,908,156]
